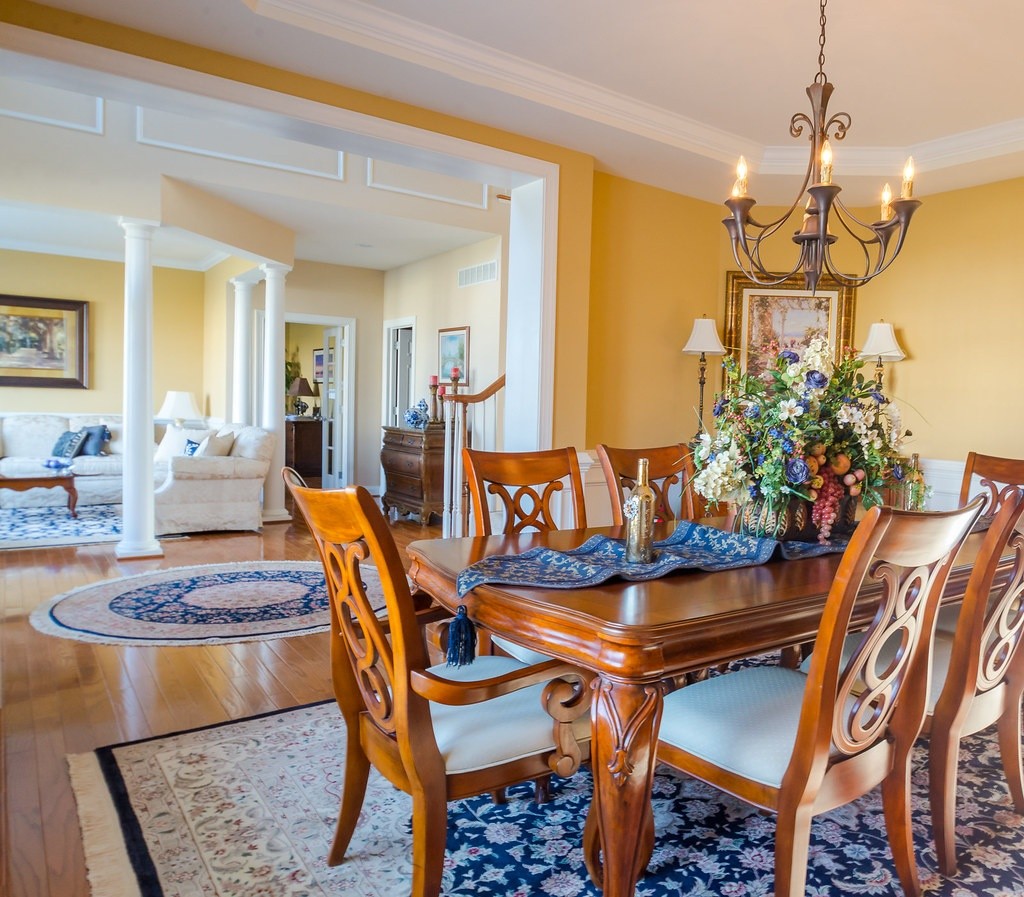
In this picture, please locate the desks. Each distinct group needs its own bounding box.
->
[403,512,1022,896]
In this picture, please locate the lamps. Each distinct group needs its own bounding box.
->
[721,0,922,295]
[681,313,727,448]
[153,390,202,431]
[286,376,314,415]
[854,317,905,429]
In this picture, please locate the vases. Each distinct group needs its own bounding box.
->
[740,479,856,540]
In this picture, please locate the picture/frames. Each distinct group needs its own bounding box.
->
[722,270,858,422]
[312,348,335,383]
[437,326,470,388]
[0,294,89,389]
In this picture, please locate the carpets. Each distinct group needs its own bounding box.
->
[0,506,187,551]
[29,560,413,647]
[63,637,1021,897]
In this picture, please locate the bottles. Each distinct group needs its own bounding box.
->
[626,458,655,562]
[404,398,429,430]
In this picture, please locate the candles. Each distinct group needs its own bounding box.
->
[430,374,437,386]
[450,367,459,378]
[437,385,446,396]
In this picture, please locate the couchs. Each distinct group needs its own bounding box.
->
[0,412,158,497]
[152,424,281,533]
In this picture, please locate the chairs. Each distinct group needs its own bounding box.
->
[282,439,1023,897]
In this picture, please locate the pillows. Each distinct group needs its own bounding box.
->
[43,422,236,470]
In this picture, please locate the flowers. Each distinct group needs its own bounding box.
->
[672,326,934,545]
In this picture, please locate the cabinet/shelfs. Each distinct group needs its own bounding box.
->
[379,426,471,526]
[285,421,332,475]
